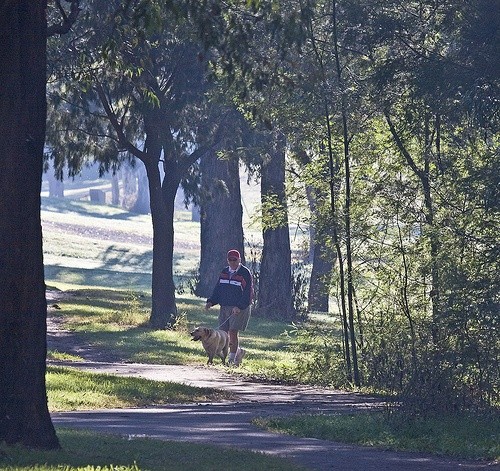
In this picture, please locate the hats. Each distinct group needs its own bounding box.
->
[227,250,240,258]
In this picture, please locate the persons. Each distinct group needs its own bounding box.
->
[206,249,255,366]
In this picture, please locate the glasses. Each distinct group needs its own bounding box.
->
[228,258,237,261]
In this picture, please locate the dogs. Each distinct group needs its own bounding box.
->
[189,326,230,367]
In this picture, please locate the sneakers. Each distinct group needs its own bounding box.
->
[225,358,234,367]
